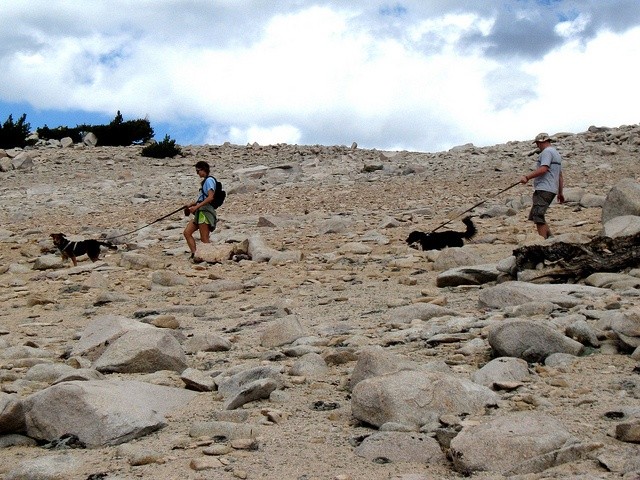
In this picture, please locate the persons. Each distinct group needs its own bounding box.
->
[519,133,565,239]
[183,161,217,259]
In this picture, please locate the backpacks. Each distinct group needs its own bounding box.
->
[199,176,226,209]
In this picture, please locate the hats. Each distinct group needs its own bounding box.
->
[532,133,557,144]
[193,161,210,172]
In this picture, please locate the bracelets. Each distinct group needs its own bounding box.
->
[526,176,529,181]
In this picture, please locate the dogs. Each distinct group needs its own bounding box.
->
[405,216,477,251]
[49,232,117,268]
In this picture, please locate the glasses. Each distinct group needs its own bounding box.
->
[196,169,203,174]
